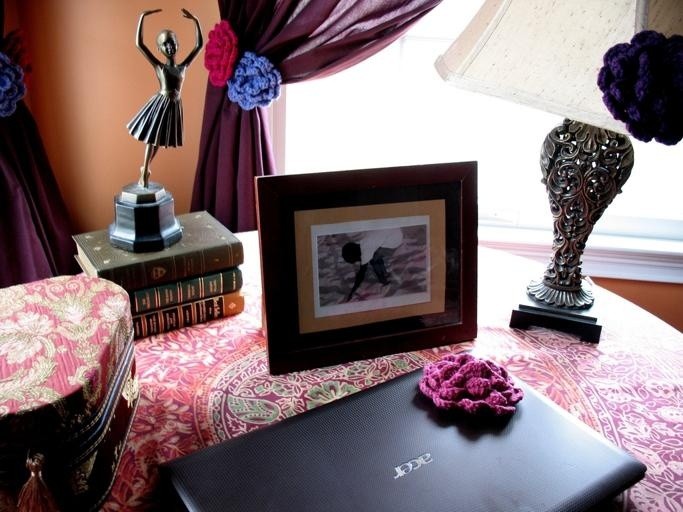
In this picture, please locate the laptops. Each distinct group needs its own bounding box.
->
[157,349,648,512]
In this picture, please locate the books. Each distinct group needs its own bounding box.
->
[71,210,244,341]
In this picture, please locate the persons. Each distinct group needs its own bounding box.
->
[126,8,203,189]
[340,227,404,303]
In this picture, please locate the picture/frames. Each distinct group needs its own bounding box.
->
[256,161,477,377]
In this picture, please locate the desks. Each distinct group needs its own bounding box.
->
[99,228,683,510]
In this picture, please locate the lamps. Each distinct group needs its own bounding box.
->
[432,0,683,343]
